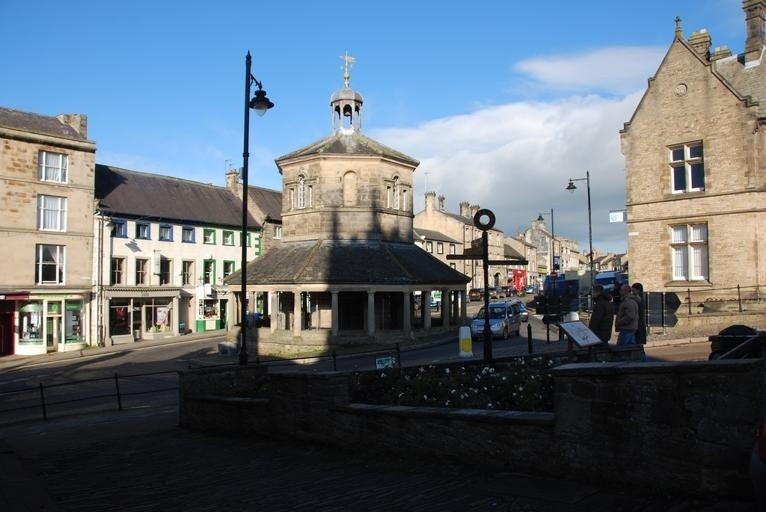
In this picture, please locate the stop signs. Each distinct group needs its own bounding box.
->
[549,270,557,281]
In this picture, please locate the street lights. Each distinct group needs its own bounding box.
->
[566,170,595,305]
[234,42,275,365]
[536,207,559,299]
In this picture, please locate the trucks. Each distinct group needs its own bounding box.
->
[596,267,628,295]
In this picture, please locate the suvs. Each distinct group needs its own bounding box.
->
[469,300,523,340]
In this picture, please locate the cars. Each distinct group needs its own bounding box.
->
[412,281,540,309]
[508,298,531,322]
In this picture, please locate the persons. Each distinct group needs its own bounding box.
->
[156,308,167,324]
[587,283,614,346]
[615,284,650,363]
[631,282,647,355]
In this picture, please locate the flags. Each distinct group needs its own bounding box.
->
[553,256,560,270]
